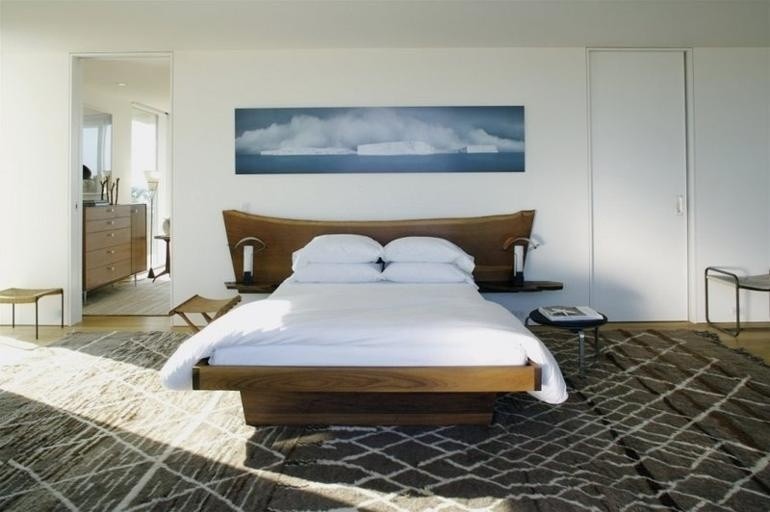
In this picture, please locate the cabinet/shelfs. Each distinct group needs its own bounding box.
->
[83,204,147,306]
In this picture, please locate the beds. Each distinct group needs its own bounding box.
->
[159,207,569,426]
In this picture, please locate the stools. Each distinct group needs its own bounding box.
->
[704,266,770,338]
[0,287,64,340]
[167,295,242,334]
[524,307,607,375]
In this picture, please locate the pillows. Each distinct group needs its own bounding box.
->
[292,264,383,283]
[380,236,477,275]
[380,261,480,289]
[292,233,383,272]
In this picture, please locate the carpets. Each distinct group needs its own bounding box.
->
[0,322,770,512]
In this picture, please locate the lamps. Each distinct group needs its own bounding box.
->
[145,170,161,277]
[513,244,525,284]
[243,245,254,286]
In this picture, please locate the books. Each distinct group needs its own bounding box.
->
[83,200,107,204]
[84,203,110,207]
[537,305,604,322]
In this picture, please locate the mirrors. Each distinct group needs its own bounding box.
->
[83,106,114,204]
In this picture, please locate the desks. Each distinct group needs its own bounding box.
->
[477,281,563,293]
[152,236,171,283]
[224,282,274,293]
